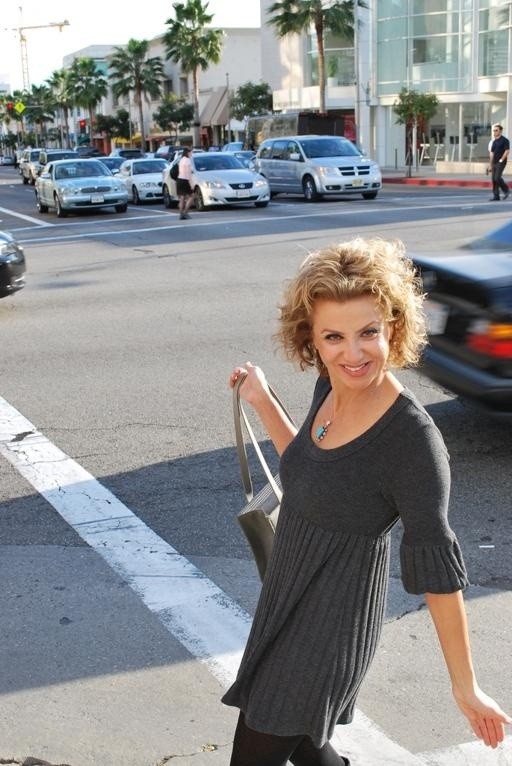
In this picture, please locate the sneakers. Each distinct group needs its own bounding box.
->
[178,213,191,220]
[488,192,510,201]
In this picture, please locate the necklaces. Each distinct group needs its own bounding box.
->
[311,384,372,446]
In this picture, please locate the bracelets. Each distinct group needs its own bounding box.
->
[499,158,503,162]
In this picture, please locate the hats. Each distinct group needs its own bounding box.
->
[182,146,193,154]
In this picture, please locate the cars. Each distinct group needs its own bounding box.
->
[14,131,256,185]
[391,215,512,421]
[1,156,14,165]
[33,158,129,218]
[113,158,172,205]
[161,151,271,211]
[0,229,27,299]
[0,155,4,166]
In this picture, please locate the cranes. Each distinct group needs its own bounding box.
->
[3,4,72,96]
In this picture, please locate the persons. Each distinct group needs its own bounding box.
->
[225,232,512,766]
[175,146,199,220]
[487,123,511,202]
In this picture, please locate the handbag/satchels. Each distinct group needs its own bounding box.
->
[169,162,179,180]
[232,371,298,588]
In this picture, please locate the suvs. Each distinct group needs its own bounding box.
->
[256,134,383,203]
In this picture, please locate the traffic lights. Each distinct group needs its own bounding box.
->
[6,101,14,110]
[79,118,88,134]
